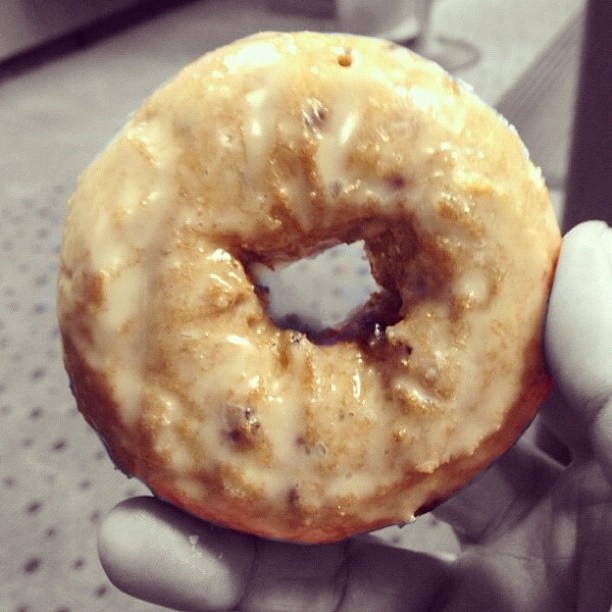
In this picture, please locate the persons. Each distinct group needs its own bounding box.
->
[95,218,611,611]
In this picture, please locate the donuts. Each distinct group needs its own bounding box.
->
[55,33,564,547]
